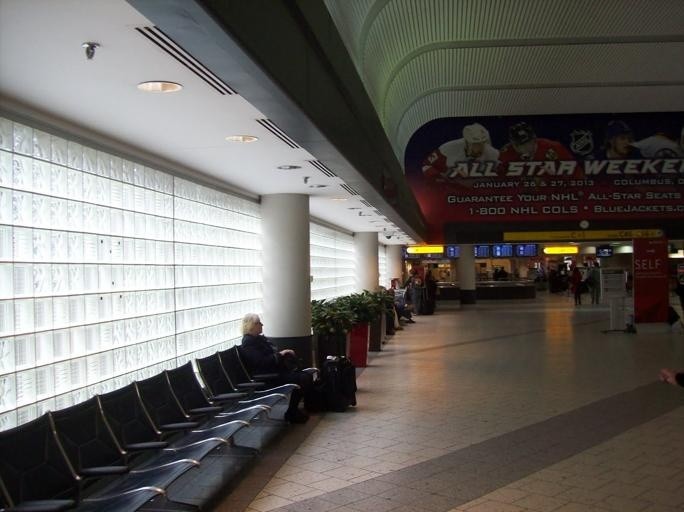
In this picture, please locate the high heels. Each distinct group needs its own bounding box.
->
[282,407,309,427]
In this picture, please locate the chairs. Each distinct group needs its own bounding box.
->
[0,345,316,512]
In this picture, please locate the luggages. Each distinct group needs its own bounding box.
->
[318,354,356,408]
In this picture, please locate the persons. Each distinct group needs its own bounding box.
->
[241,311,326,427]
[424,269,437,307]
[584,262,600,304]
[569,267,582,305]
[662,273,684,312]
[419,122,500,180]
[498,120,585,178]
[386,287,415,325]
[657,366,683,388]
[492,269,499,281]
[594,120,644,159]
[497,266,508,281]
[633,116,682,158]
[399,267,420,315]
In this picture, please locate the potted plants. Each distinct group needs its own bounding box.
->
[311,286,396,368]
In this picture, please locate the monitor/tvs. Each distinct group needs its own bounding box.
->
[472,245,490,258]
[491,244,513,258]
[596,246,613,257]
[446,245,461,259]
[515,244,537,258]
